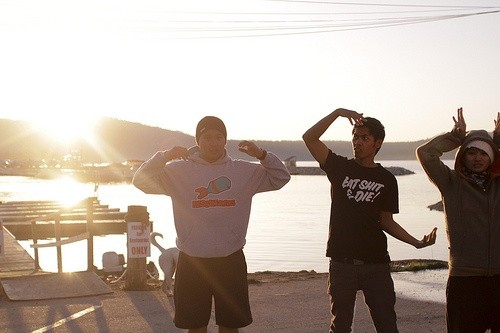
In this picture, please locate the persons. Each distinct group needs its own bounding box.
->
[417,107,500,332]
[133,116,290,333]
[303,108,438,333]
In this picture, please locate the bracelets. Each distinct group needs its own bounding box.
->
[257,149,267,161]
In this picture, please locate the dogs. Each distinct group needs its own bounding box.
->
[150,232,180,297]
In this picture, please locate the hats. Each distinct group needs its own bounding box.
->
[196,116,227,146]
[463,140,494,164]
